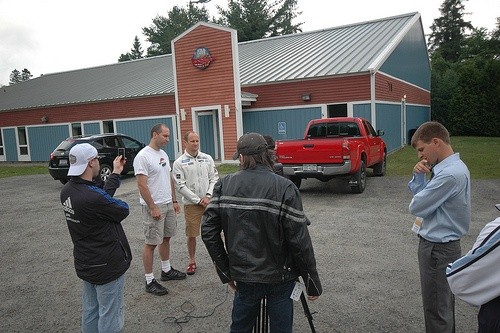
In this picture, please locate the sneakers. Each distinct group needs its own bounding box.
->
[161,265,186,281]
[145,279,169,296]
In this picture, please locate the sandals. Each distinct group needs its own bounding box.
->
[186,261,197,275]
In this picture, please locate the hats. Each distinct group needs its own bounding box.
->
[66,143,98,177]
[233,132,269,162]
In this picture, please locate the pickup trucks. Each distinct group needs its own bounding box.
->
[274,117,387,194]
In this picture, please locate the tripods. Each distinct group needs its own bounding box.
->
[254,277,316,333]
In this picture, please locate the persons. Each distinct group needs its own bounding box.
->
[409,122,470,333]
[263,135,285,178]
[133,124,186,295]
[172,131,219,274]
[61,143,133,333]
[446,217,500,333]
[201,133,322,333]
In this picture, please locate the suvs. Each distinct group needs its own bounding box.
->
[48,133,146,185]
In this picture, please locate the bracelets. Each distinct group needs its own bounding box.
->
[172,200,179,204]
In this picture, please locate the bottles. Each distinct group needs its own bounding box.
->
[411,217,423,234]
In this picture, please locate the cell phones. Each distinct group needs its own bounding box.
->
[118,148,125,160]
